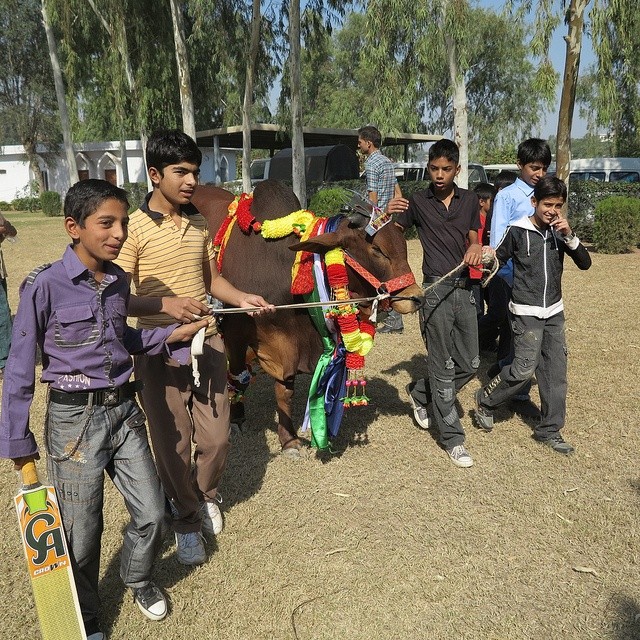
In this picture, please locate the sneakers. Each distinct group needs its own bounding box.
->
[175,531,207,566]
[406,381,432,429]
[447,445,473,468]
[198,501,223,534]
[124,582,167,621]
[545,436,574,454]
[473,387,493,432]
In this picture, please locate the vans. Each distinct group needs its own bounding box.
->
[547,157,640,221]
[250,158,272,185]
[360,162,488,189]
[468,164,521,186]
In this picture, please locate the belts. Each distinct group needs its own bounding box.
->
[49,380,146,406]
[423,275,471,288]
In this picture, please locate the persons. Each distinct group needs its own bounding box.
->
[112,130,276,565]
[384,139,483,468]
[358,127,405,337]
[468,183,501,355]
[0,179,172,640]
[484,171,530,356]
[485,139,552,401]
[0,209,17,377]
[473,175,592,453]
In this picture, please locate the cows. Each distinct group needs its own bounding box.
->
[190,179,425,454]
